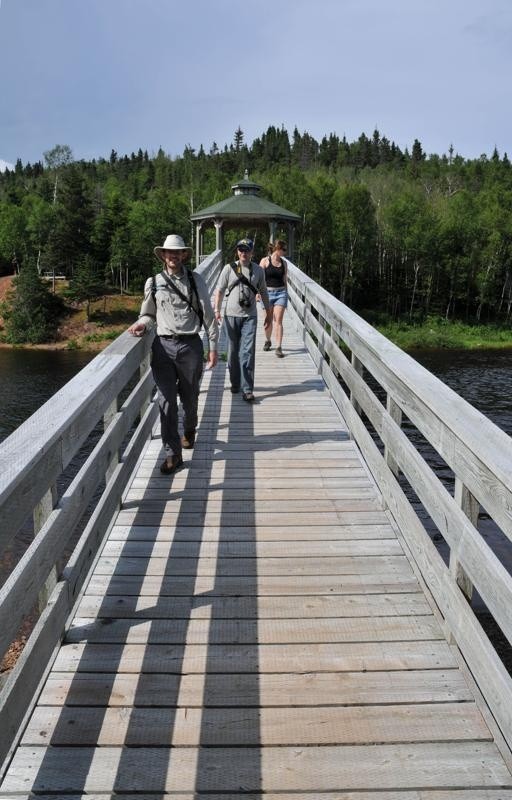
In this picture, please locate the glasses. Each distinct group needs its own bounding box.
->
[237,248,252,252]
[280,247,287,252]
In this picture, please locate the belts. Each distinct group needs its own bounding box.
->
[156,333,199,339]
[267,287,286,292]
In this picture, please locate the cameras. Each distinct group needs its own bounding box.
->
[239,297,249,307]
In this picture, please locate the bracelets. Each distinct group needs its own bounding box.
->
[214,310,220,312]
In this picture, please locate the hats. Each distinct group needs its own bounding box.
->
[154,235,193,264]
[237,238,253,250]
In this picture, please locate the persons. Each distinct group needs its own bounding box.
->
[128,234,219,474]
[255,239,288,358]
[214,238,272,401]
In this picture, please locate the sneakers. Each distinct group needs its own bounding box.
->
[231,384,241,394]
[262,341,272,351]
[242,391,254,401]
[275,347,284,358]
[181,427,195,449]
[160,454,183,475]
